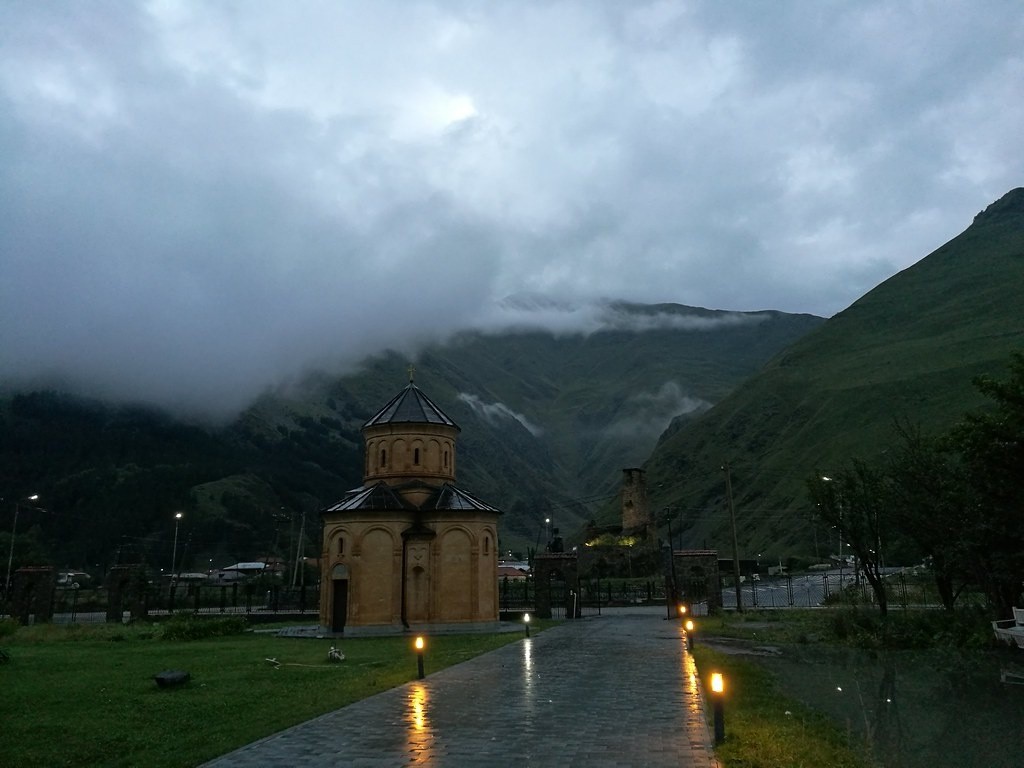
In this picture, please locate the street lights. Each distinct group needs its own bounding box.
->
[168,513,182,611]
[2,493,39,615]
[545,518,550,543]
[822,476,844,570]
